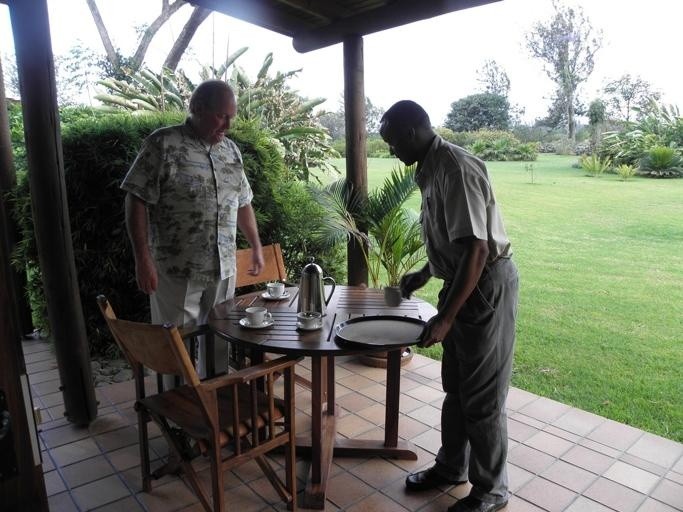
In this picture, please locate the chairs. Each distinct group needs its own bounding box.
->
[236,242,289,296]
[93,293,306,512]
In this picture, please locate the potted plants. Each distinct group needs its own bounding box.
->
[306,160,431,369]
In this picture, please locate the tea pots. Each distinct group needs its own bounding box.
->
[296,256,336,318]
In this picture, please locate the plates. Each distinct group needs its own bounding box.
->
[261,291,290,300]
[239,317,274,328]
[295,322,324,331]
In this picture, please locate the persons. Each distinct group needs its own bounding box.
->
[119,80,264,462]
[377,100,522,512]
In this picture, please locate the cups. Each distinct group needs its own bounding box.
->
[266,282,285,297]
[296,310,321,328]
[245,306,272,325]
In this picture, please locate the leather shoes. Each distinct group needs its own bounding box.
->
[407,468,467,491]
[448,494,509,512]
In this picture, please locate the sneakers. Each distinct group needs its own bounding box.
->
[166,428,201,459]
